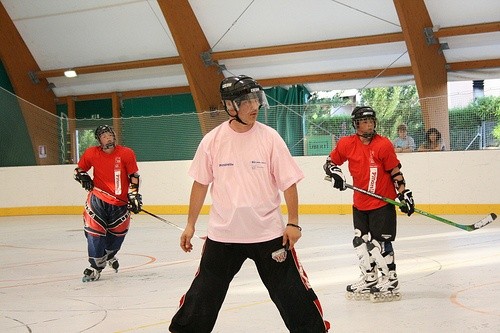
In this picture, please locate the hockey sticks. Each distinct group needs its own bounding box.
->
[93,186,208,241]
[323,175,498,233]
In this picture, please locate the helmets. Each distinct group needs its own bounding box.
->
[219,75,262,100]
[95,125,116,144]
[350,106,377,121]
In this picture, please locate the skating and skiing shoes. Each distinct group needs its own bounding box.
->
[107,256,119,273]
[81,267,103,282]
[370,270,401,303]
[345,272,379,301]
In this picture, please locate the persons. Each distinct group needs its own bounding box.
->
[73,125,142,282]
[394,125,416,152]
[323,106,414,302]
[417,128,446,151]
[169,75,330,333]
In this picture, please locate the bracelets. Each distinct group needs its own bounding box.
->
[287,223,302,231]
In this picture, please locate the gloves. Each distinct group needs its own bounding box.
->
[127,194,143,214]
[328,166,347,192]
[397,189,415,216]
[74,168,94,192]
